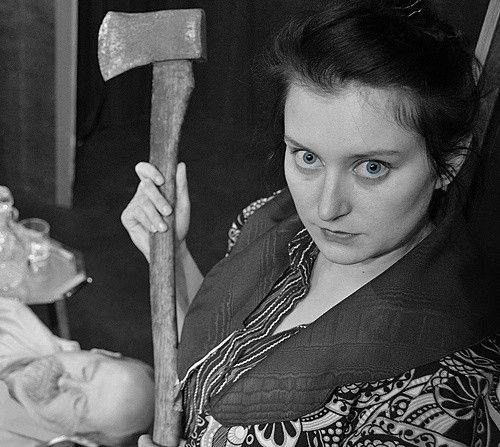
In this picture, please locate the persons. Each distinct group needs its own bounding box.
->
[119,0,500,447]
[0,293,156,447]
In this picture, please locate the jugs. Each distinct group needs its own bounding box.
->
[0,185,27,296]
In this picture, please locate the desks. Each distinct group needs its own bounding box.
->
[2,228,88,339]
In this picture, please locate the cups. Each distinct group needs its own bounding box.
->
[19,216,51,272]
